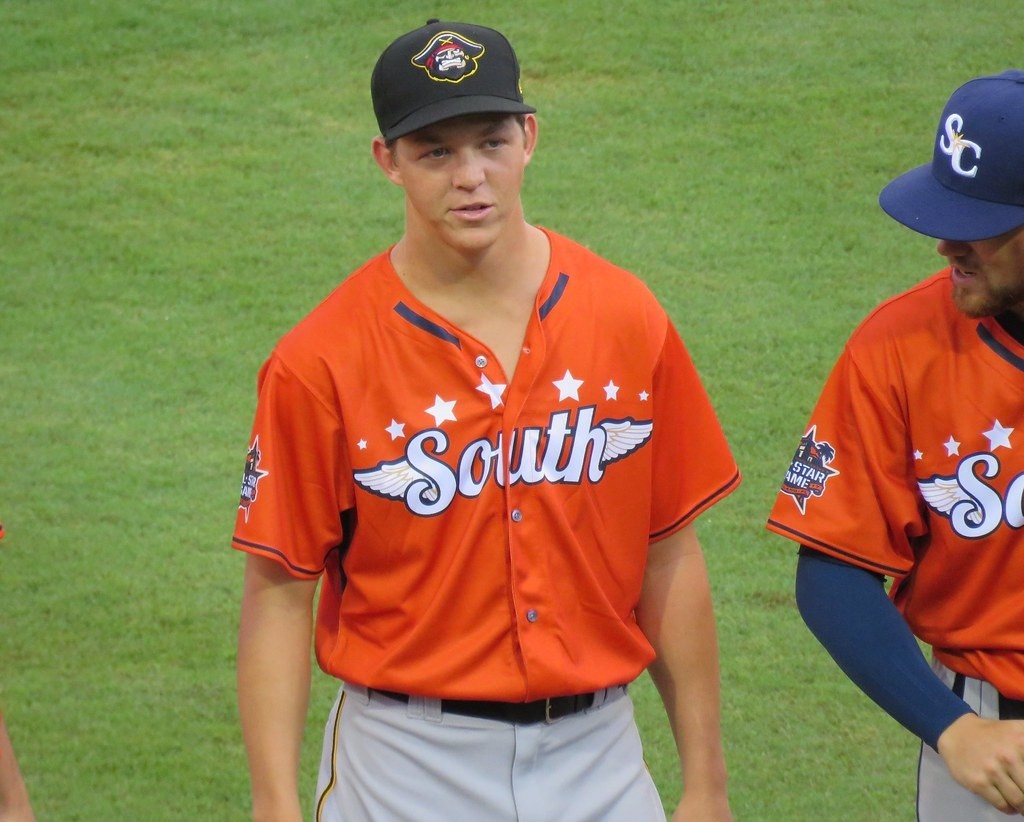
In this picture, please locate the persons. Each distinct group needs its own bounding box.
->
[0,523,36,822]
[764,69,1024,821]
[231,20,743,822]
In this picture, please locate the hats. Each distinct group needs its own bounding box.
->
[879,69,1024,241]
[371,18,537,139]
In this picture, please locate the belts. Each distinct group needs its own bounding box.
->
[368,684,627,725]
[952,672,1024,720]
